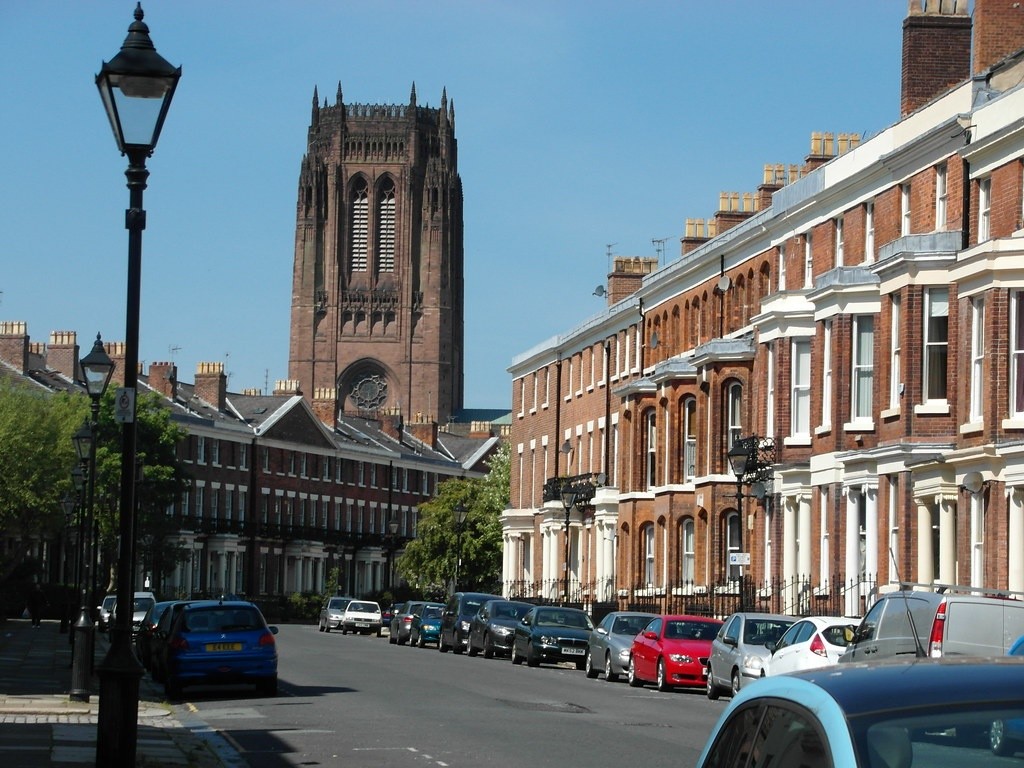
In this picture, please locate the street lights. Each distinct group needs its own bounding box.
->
[453,500,469,591]
[560,481,578,607]
[95,0,182,768]
[61,416,92,641]
[70,331,116,701]
[727,435,751,613]
[389,516,400,605]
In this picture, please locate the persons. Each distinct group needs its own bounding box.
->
[25,572,49,628]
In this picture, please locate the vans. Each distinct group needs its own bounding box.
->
[838,582,1023,742]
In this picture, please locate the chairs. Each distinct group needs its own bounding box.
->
[617,621,629,633]
[745,624,758,643]
[190,615,208,633]
[563,616,575,626]
[669,624,678,638]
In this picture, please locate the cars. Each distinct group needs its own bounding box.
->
[706,612,802,700]
[759,615,865,677]
[984,635,1023,757]
[628,614,726,693]
[467,600,537,659]
[438,592,506,654]
[585,612,657,682]
[318,597,425,645]
[695,657,1023,768]
[96,592,278,701]
[410,603,447,650]
[511,606,595,668]
